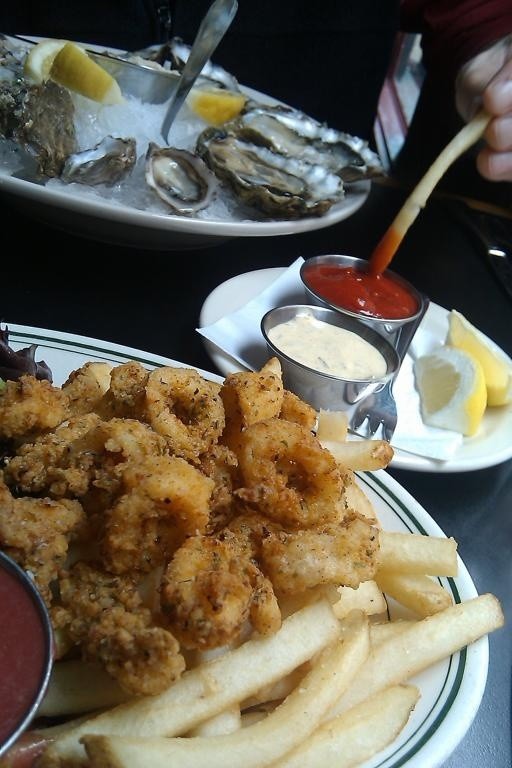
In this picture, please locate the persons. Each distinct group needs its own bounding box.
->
[1,1,511,184]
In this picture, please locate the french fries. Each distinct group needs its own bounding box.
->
[1,407,506,768]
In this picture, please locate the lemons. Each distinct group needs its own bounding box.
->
[21,37,87,84]
[48,44,123,108]
[446,312,512,408]
[183,87,247,129]
[412,345,488,439]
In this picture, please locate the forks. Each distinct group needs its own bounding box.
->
[350,291,431,442]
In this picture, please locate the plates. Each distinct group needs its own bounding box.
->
[1,29,376,234]
[0,322,491,768]
[198,266,512,474]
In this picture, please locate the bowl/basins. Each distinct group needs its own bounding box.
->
[258,304,400,416]
[0,546,56,756]
[298,254,424,339]
[82,50,247,126]
